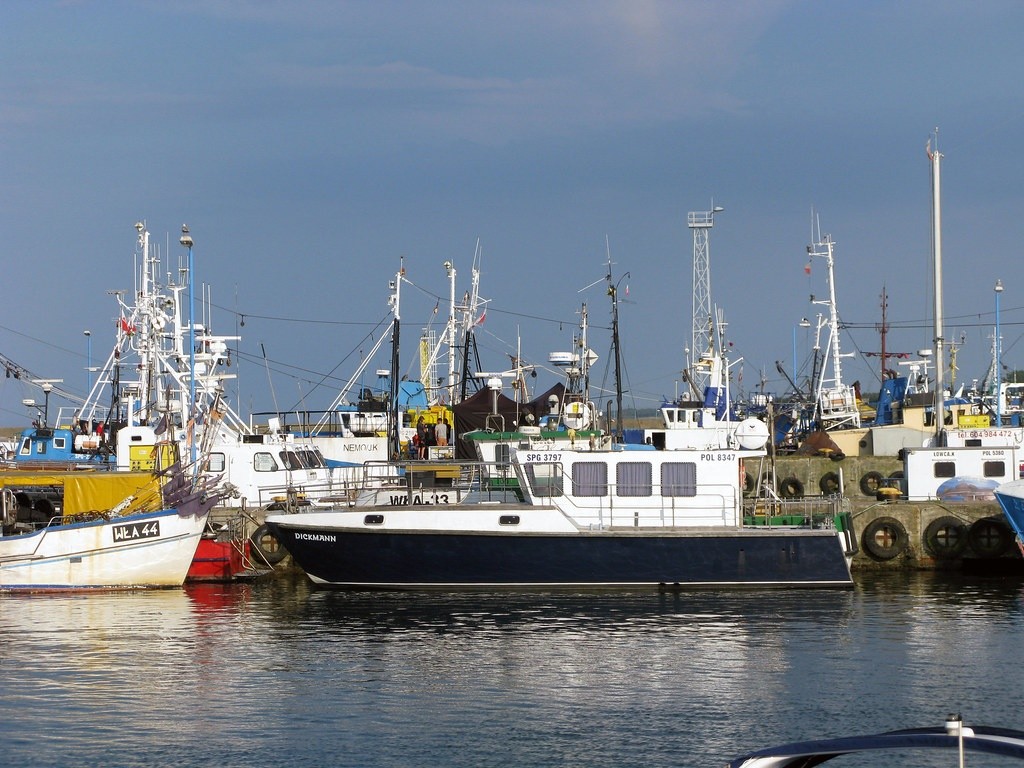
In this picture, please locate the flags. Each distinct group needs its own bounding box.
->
[121,311,136,333]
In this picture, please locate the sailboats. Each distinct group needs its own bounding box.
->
[1,129,1023,597]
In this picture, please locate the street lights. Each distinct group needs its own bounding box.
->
[792,322,809,403]
[84,330,92,418]
[180,222,199,491]
[993,279,1003,426]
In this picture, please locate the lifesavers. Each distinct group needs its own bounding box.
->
[817,470,848,499]
[247,521,293,568]
[740,470,805,503]
[859,516,912,561]
[922,514,1018,566]
[860,470,906,498]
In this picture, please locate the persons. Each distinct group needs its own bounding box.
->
[96,421,105,439]
[416,417,451,461]
